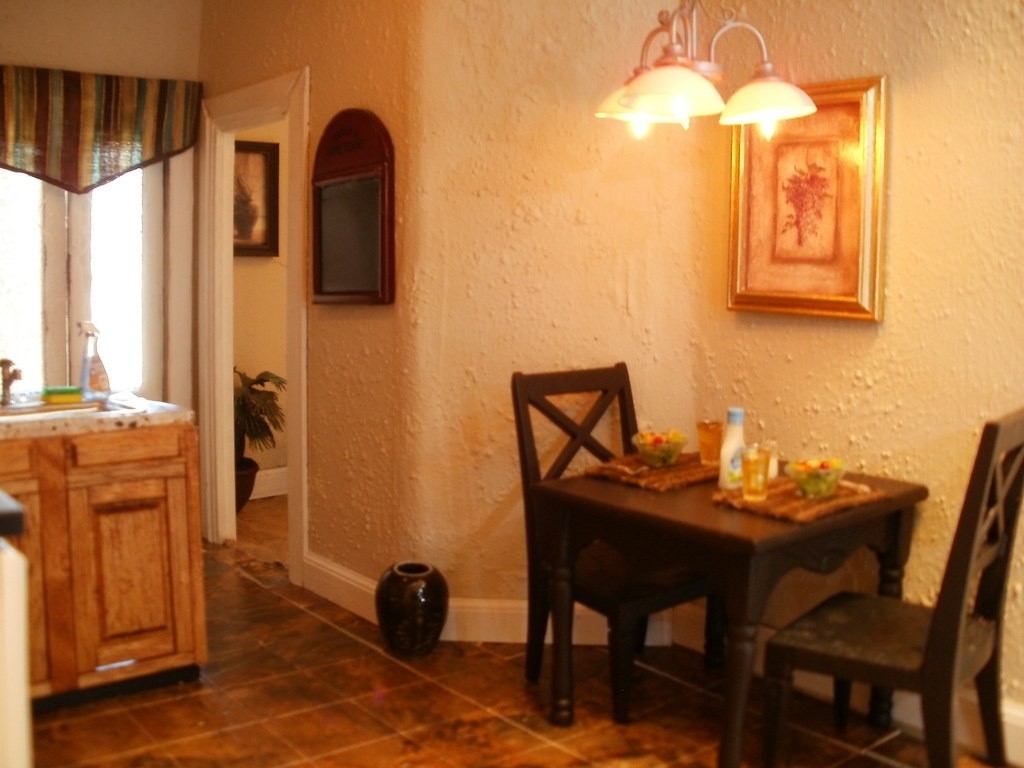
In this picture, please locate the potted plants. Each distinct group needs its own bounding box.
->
[233,366,286,509]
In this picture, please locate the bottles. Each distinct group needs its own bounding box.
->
[718,406,744,494]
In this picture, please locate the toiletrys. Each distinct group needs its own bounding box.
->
[76,322,111,402]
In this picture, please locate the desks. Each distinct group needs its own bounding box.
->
[538,444,930,767]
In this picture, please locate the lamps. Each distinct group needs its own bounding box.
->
[593,0,818,141]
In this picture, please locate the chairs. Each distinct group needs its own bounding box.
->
[754,406,1024,768]
[511,361,728,724]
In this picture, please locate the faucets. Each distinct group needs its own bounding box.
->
[0,358,23,406]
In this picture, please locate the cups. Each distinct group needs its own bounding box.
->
[694,418,722,468]
[741,446,770,501]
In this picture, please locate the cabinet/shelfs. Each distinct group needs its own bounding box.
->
[1,422,214,708]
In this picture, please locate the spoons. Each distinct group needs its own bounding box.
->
[594,461,648,477]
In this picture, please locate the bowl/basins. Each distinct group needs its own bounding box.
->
[633,430,684,467]
[785,459,844,499]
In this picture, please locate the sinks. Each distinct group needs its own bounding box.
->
[0,400,147,417]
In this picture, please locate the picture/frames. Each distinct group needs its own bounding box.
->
[727,71,892,324]
[234,139,279,256]
